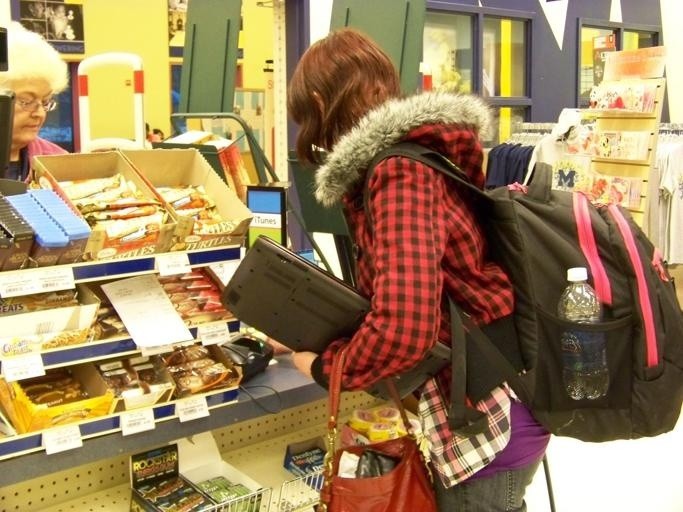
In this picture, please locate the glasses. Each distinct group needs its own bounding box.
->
[16,98,58,113]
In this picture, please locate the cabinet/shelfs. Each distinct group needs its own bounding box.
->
[550,43,665,229]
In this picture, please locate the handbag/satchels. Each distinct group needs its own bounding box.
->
[316,341,438,512]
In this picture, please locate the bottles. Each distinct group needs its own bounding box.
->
[558,265,611,402]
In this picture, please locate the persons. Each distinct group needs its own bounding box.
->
[0,20,70,183]
[19,1,78,40]
[264,28,552,510]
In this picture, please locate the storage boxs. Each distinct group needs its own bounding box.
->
[0,149,376,512]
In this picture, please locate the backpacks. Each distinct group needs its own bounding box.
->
[361,142,683,445]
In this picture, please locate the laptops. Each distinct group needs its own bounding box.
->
[219,235,452,403]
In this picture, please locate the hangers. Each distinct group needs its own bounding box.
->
[657,123,683,136]
[503,122,556,146]
